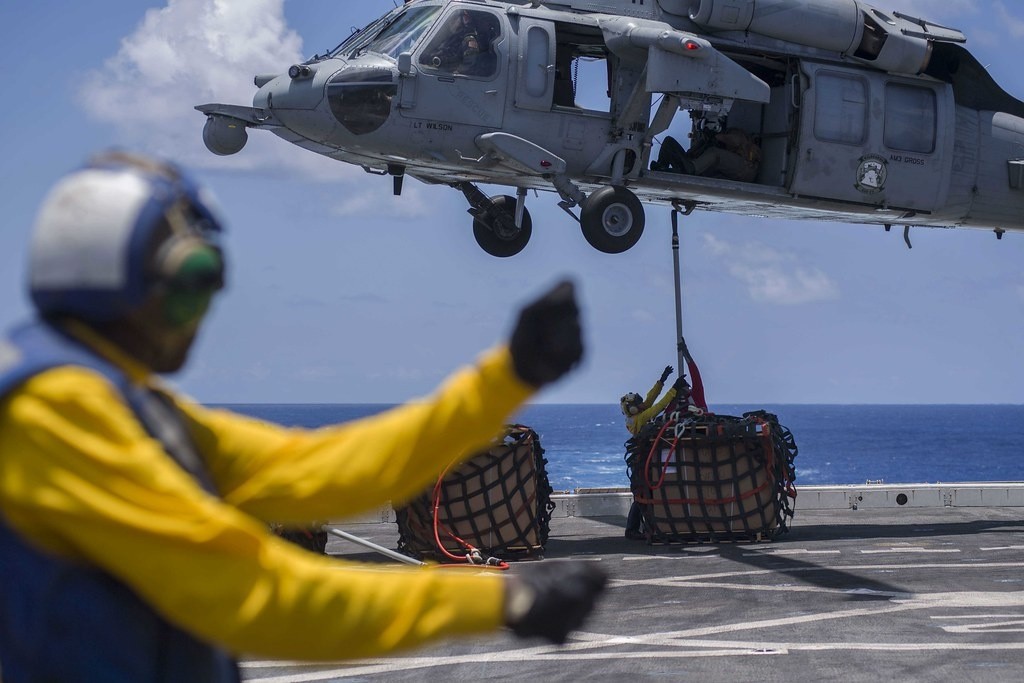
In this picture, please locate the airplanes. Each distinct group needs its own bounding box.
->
[195,0,1023,255]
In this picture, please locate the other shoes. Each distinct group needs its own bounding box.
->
[625,528,643,540]
[650,161,667,171]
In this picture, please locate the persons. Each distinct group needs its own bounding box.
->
[663,335,709,416]
[428,9,479,75]
[650,125,762,178]
[0,141,610,683]
[619,365,688,543]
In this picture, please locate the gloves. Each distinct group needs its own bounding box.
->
[660,365,674,382]
[511,282,584,388]
[672,374,687,391]
[502,559,608,644]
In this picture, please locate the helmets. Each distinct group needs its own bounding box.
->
[25,145,231,318]
[621,392,643,417]
[463,33,489,67]
